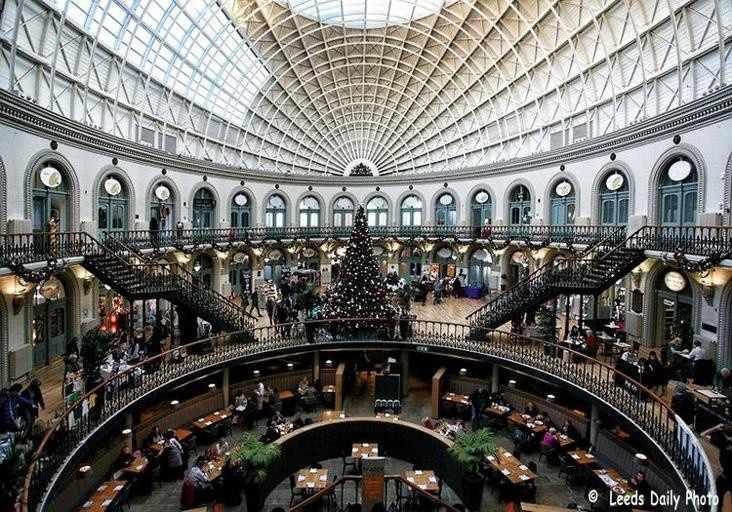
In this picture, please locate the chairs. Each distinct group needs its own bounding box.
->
[112,484,130,511]
[289,433,466,512]
[580,318,732,424]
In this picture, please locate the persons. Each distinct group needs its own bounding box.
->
[386,265,467,306]
[467,299,732,512]
[0,276,323,506]
[483,216,491,239]
[149,216,159,247]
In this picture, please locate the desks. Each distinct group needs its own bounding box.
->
[122,408,232,473]
[442,391,639,500]
[78,481,128,512]
[321,385,336,409]
[278,389,296,417]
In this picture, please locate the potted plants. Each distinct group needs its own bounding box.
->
[80,324,120,420]
